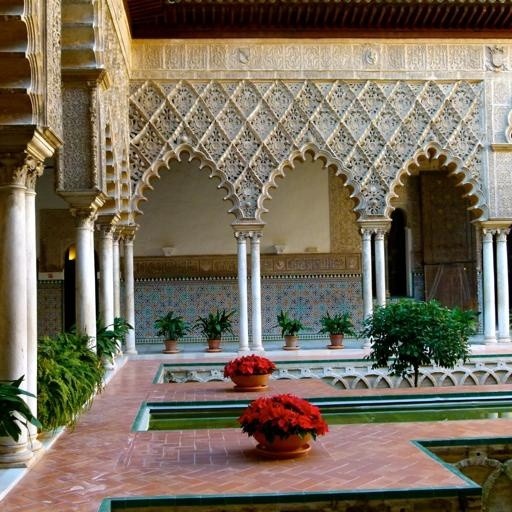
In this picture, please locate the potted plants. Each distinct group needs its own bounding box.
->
[70,317,133,373]
[37,329,104,439]
[272,309,305,347]
[317,311,357,346]
[153,311,191,351]
[193,309,236,349]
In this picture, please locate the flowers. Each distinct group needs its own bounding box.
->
[223,355,276,375]
[238,394,329,441]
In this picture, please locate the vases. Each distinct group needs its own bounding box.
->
[231,374,270,387]
[253,432,304,452]
